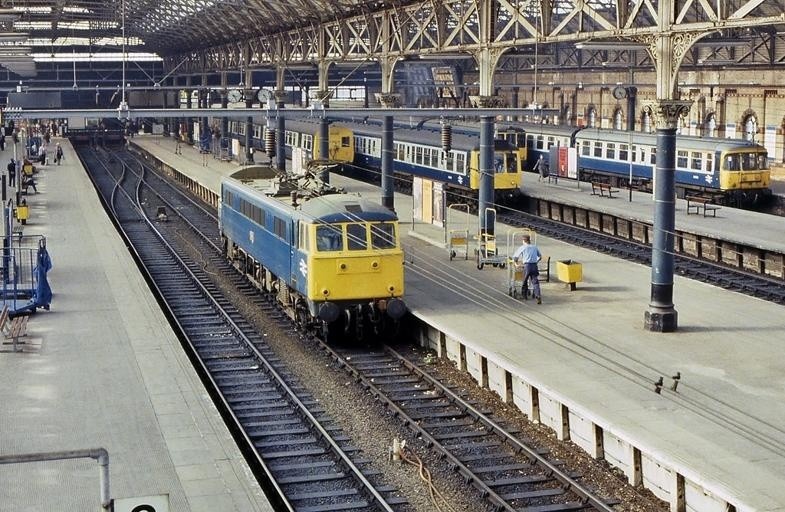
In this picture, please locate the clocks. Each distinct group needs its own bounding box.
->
[256,88,273,105]
[227,89,242,104]
[612,85,627,100]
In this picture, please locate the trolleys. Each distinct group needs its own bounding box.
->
[445,227,470,261]
[0,230,55,320]
[503,228,536,301]
[474,206,507,272]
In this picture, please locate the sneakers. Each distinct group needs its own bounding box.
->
[536,297,542,304]
[520,296,527,300]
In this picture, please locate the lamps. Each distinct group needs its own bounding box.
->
[117,1,130,124]
[153,53,161,92]
[238,62,244,88]
[72,47,79,93]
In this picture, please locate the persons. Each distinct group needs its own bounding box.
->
[0,120,63,194]
[494,159,502,173]
[533,153,549,183]
[512,235,543,304]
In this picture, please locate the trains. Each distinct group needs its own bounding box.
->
[218,157,410,346]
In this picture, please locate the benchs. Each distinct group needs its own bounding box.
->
[590,181,620,199]
[5,181,28,246]
[685,195,723,218]
[1,303,30,353]
